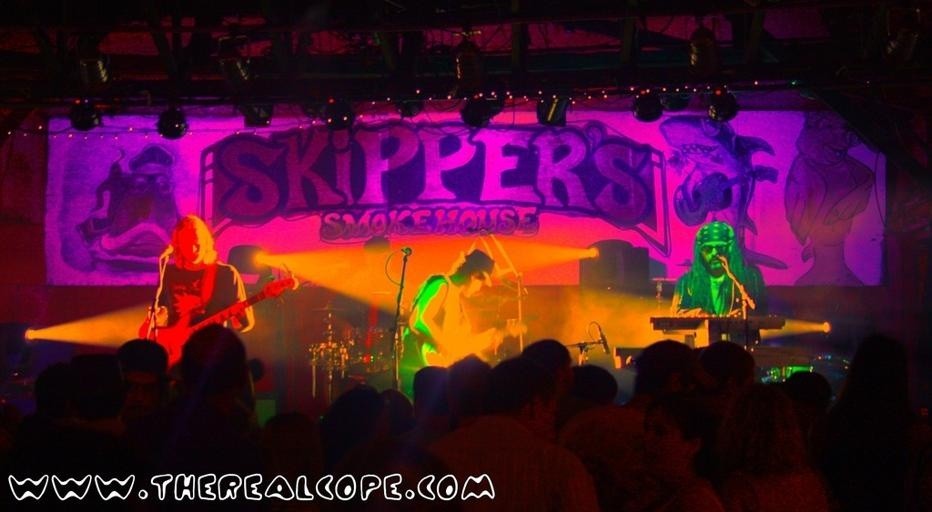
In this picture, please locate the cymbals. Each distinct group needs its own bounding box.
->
[312,307,345,312]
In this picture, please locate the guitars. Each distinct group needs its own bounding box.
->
[150,273,297,368]
[421,319,528,368]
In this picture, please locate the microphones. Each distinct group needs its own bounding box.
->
[599,332,611,354]
[401,247,413,256]
[159,243,174,258]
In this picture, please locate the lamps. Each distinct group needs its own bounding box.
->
[67,85,743,138]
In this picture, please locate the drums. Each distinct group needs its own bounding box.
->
[309,330,397,373]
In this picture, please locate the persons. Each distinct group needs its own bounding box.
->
[139,214,255,371]
[668,220,770,344]
[0,321,932,512]
[397,248,508,407]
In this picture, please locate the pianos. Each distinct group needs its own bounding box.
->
[650,316,785,334]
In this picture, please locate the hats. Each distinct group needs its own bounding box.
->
[695,220,736,249]
[462,248,496,289]
